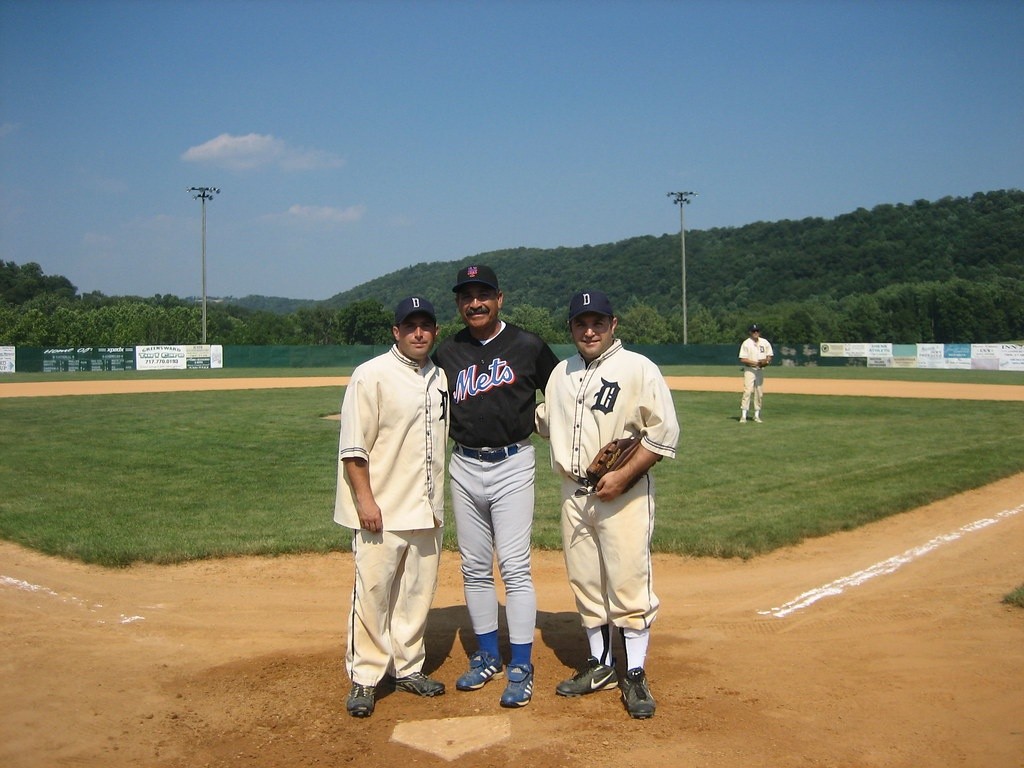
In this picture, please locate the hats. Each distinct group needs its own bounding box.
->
[394,297,437,327]
[749,324,758,331]
[569,292,614,326]
[451,265,497,295]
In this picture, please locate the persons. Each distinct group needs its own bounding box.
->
[333,297,450,717]
[431,266,560,708]
[737,323,774,422]
[535,290,680,720]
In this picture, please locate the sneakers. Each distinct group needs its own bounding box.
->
[555,656,619,698]
[455,649,503,690]
[753,417,764,424]
[739,416,748,424]
[499,662,534,707]
[387,672,445,696]
[346,681,375,718]
[621,668,656,718]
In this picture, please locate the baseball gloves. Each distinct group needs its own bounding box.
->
[587,437,663,495]
[758,359,771,368]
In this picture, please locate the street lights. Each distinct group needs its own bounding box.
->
[187,187,220,345]
[665,190,701,347]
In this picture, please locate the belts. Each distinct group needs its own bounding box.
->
[453,442,518,462]
